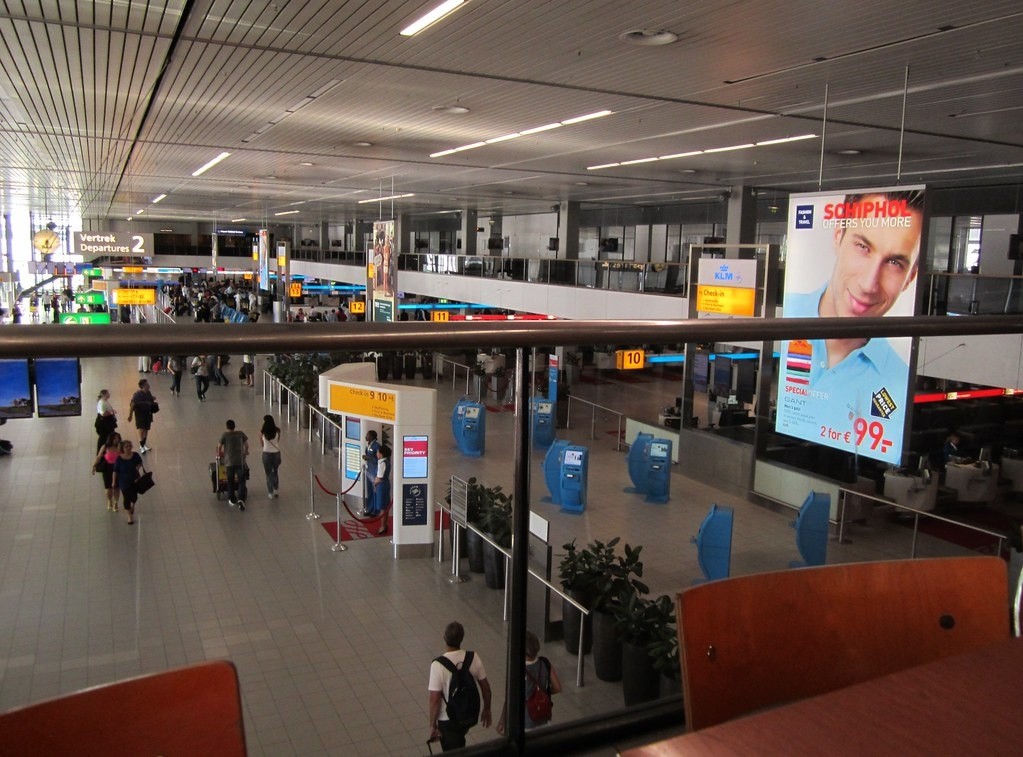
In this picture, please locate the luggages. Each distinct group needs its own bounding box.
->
[178,306,189,316]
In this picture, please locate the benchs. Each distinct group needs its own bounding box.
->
[664,416,699,430]
[992,428,1023,458]
[871,450,919,495]
[936,431,976,455]
[719,408,752,427]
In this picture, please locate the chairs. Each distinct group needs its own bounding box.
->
[0,658,249,757]
[677,553,1012,735]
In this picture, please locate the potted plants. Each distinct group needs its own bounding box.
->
[266,348,433,458]
[444,475,513,590]
[473,361,506,400]
[590,542,649,683]
[536,353,579,427]
[554,535,620,657]
[645,634,683,699]
[618,593,673,708]
[707,388,722,427]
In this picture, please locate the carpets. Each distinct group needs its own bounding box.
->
[322,510,452,541]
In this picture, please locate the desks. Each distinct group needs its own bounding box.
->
[712,408,722,429]
[882,467,940,518]
[600,634,1023,757]
[944,460,999,516]
[658,411,682,428]
[769,457,875,527]
[1001,455,1023,504]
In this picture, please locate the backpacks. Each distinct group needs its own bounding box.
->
[183,294,188,303]
[525,662,552,724]
[433,650,480,728]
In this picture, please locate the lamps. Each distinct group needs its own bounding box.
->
[913,387,1023,403]
[400,0,467,36]
[768,205,779,215]
[34,187,61,254]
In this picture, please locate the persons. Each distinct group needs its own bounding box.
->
[940,435,965,459]
[495,631,562,737]
[0,276,508,536]
[429,622,491,752]
[374,231,393,297]
[774,189,926,465]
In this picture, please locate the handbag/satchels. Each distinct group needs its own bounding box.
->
[100,415,117,428]
[96,456,107,472]
[239,367,246,380]
[191,366,199,373]
[151,402,159,413]
[135,471,154,494]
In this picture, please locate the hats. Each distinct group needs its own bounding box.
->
[526,631,539,657]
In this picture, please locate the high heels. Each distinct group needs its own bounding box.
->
[377,525,388,534]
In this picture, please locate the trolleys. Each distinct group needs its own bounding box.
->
[208,452,249,500]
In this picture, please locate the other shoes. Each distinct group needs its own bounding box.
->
[228,499,235,506]
[140,445,151,454]
[171,391,180,398]
[198,394,206,403]
[127,510,134,525]
[242,382,254,388]
[215,380,229,385]
[364,513,376,518]
[269,490,279,499]
[108,500,119,512]
[238,501,245,510]
